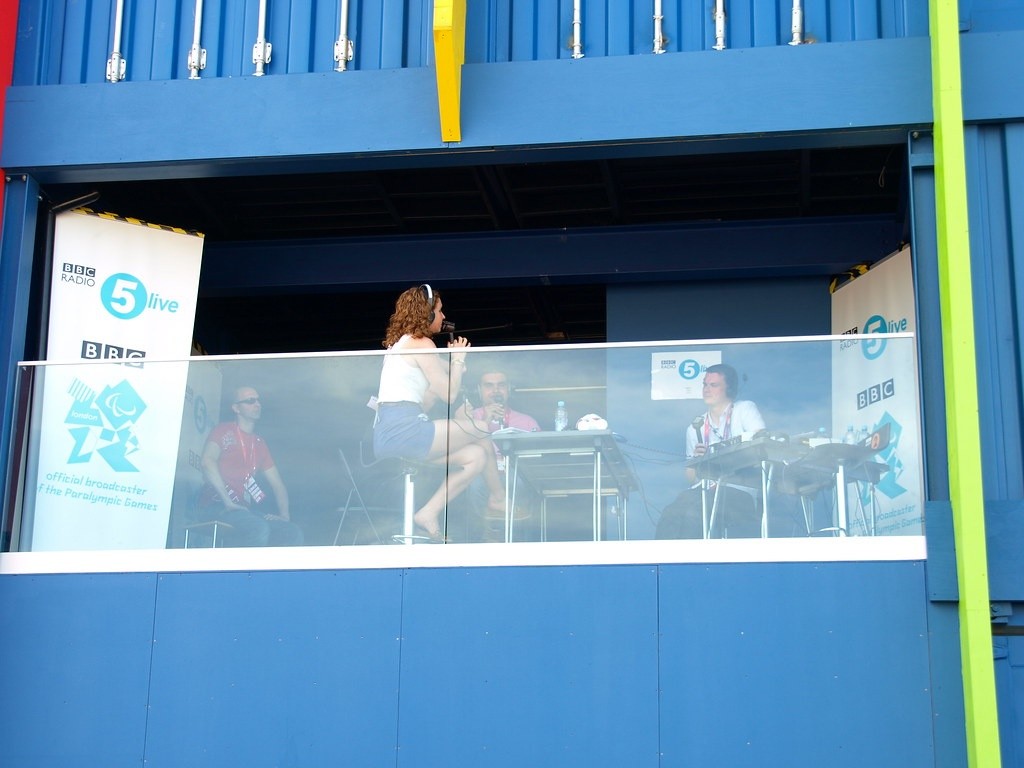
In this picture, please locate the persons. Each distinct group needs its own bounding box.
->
[655,364,767,540]
[456,363,543,542]
[373,286,532,542]
[197,387,304,547]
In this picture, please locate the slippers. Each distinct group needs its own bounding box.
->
[414,527,451,541]
[484,504,532,520]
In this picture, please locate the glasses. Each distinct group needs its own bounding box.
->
[235,397,264,404]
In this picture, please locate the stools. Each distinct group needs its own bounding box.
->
[179,491,237,548]
[356,440,465,544]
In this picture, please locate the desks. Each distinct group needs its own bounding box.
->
[684,439,889,539]
[492,428,638,543]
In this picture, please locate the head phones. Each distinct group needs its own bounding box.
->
[727,367,739,397]
[476,371,512,404]
[418,284,435,325]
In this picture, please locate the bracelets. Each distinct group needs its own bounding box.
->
[451,357,466,371]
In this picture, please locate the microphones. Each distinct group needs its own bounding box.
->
[494,395,504,431]
[441,322,455,344]
[692,416,704,445]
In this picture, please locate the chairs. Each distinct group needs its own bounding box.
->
[332,446,399,545]
[784,422,890,538]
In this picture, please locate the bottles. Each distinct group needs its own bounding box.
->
[842,424,857,445]
[555,401,568,432]
[817,427,831,447]
[858,424,869,441]
